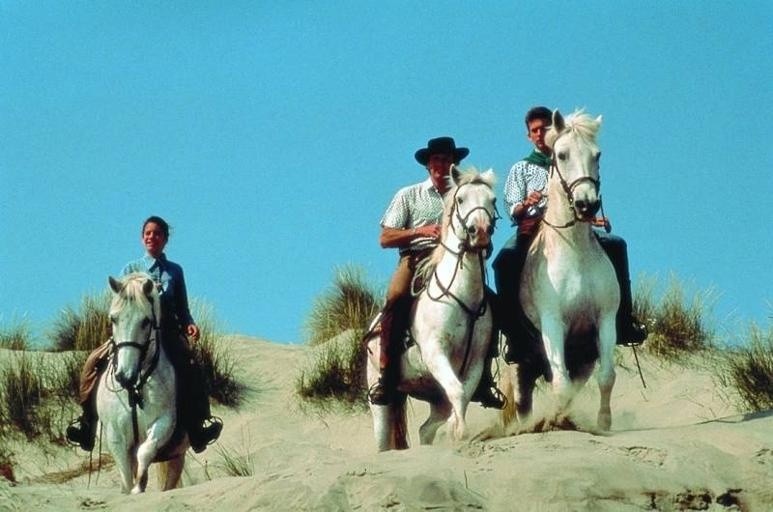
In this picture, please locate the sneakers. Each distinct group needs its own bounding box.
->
[471,386,503,408]
[617,326,644,343]
[371,375,395,404]
[67,417,96,451]
[504,338,527,361]
[188,422,222,453]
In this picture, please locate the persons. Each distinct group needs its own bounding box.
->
[492,108,646,361]
[66,216,222,453]
[372,137,498,408]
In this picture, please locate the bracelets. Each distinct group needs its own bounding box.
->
[522,203,528,209]
[414,228,416,238]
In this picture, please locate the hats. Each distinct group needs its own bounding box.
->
[415,137,469,165]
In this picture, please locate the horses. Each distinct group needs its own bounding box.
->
[90,270,191,497]
[362,162,499,454]
[515,105,624,432]
